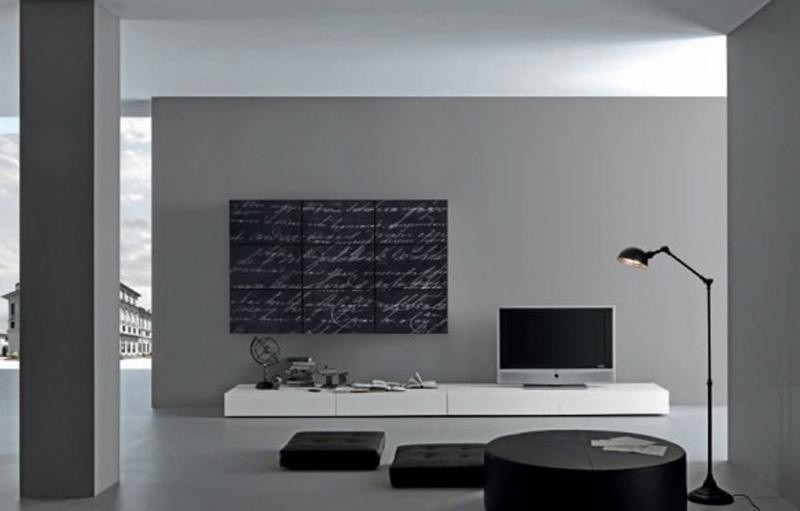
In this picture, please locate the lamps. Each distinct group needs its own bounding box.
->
[616,246,735,505]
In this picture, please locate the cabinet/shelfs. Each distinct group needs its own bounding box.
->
[224,383,671,417]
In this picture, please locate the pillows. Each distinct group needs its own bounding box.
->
[389,443,487,488]
[279,432,385,470]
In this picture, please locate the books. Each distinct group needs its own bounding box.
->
[350,371,439,392]
[284,360,317,387]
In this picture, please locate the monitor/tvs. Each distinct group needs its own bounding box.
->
[497,305,615,388]
